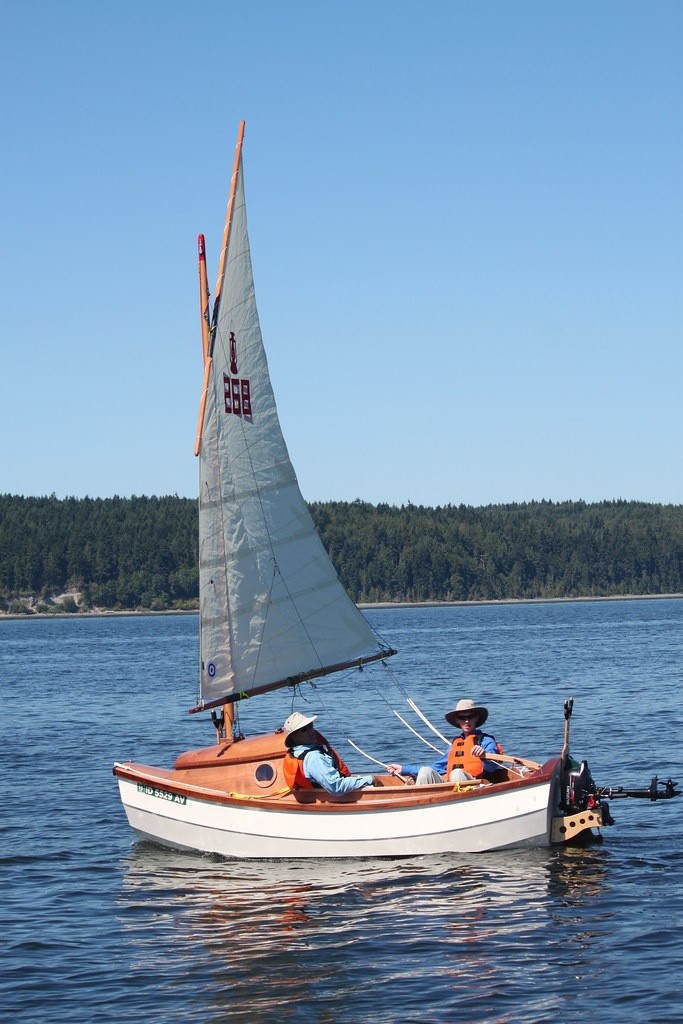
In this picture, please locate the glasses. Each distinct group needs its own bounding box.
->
[301,724,313,732]
[458,714,477,720]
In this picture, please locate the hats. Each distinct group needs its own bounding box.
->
[445,699,489,729]
[284,712,318,748]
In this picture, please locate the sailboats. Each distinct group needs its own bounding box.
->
[112,119,615,858]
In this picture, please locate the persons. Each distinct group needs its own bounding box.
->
[385,699,503,785]
[283,712,384,795]
